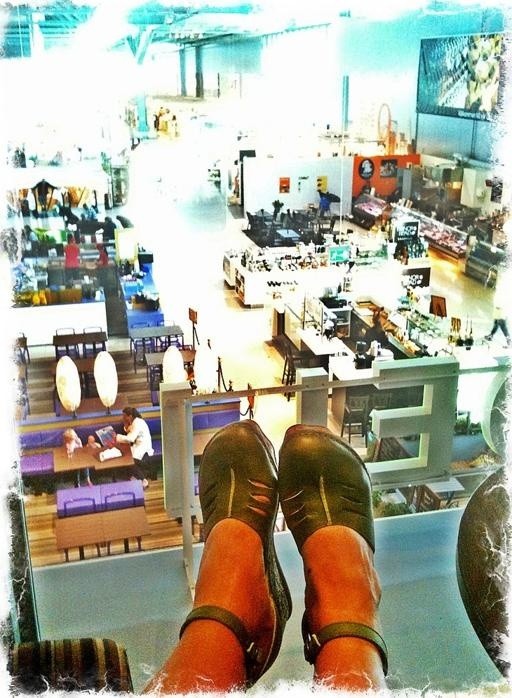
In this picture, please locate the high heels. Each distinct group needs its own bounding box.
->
[179,418,291,686]
[278,424,388,671]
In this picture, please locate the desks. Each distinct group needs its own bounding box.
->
[53,427,286,563]
[271,292,490,383]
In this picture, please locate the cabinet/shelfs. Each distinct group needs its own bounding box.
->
[224,192,506,308]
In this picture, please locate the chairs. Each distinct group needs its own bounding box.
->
[13,319,198,404]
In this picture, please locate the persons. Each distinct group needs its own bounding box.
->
[465,34,501,112]
[85,435,101,449]
[363,318,388,347]
[319,192,331,216]
[484,307,511,346]
[63,429,93,488]
[24,179,133,288]
[112,407,155,488]
[138,419,391,692]
